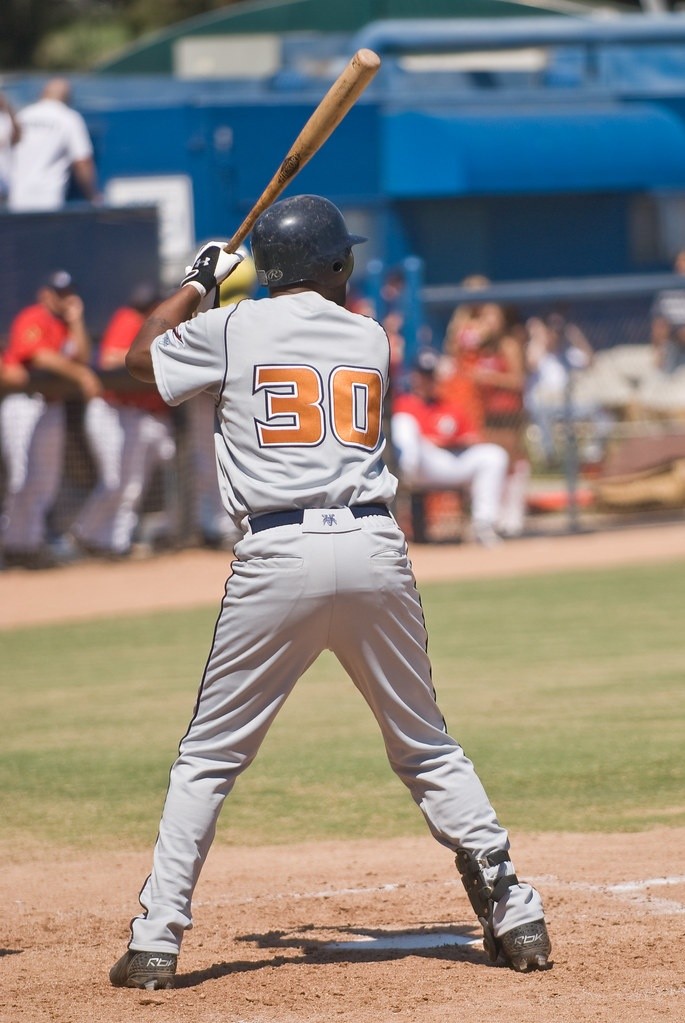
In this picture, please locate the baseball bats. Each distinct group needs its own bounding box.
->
[223,48,381,254]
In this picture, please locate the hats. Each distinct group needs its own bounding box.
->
[49,270,78,296]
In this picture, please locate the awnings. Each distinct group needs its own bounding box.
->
[379,107,685,189]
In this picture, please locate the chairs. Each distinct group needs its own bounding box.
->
[383,477,467,548]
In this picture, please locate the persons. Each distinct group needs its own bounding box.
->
[0,76,99,215]
[0,268,102,570]
[109,193,554,989]
[47,275,685,561]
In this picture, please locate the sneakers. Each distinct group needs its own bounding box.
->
[496,918,552,970]
[109,950,177,990]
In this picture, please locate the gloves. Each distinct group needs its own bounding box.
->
[180,241,245,297]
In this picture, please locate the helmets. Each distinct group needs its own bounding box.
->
[248,195,369,287]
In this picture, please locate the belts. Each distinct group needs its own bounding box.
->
[249,503,391,533]
